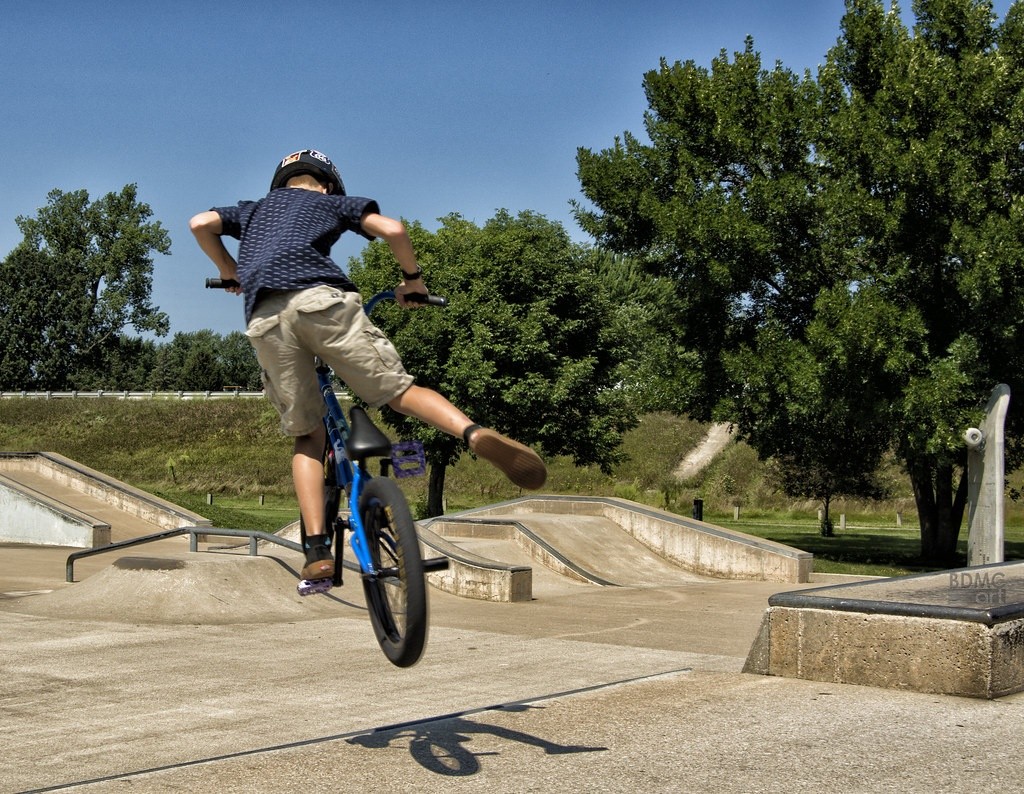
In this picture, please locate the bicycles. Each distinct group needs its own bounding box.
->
[205,277,450,669]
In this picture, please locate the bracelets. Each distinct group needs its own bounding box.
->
[402,265,422,280]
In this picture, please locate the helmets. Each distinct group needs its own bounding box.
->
[269,149,346,198]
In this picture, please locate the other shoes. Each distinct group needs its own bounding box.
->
[300,545,335,581]
[469,428,547,490]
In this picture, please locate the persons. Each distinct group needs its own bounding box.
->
[188,149,546,579]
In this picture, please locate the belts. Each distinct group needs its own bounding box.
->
[252,281,358,307]
